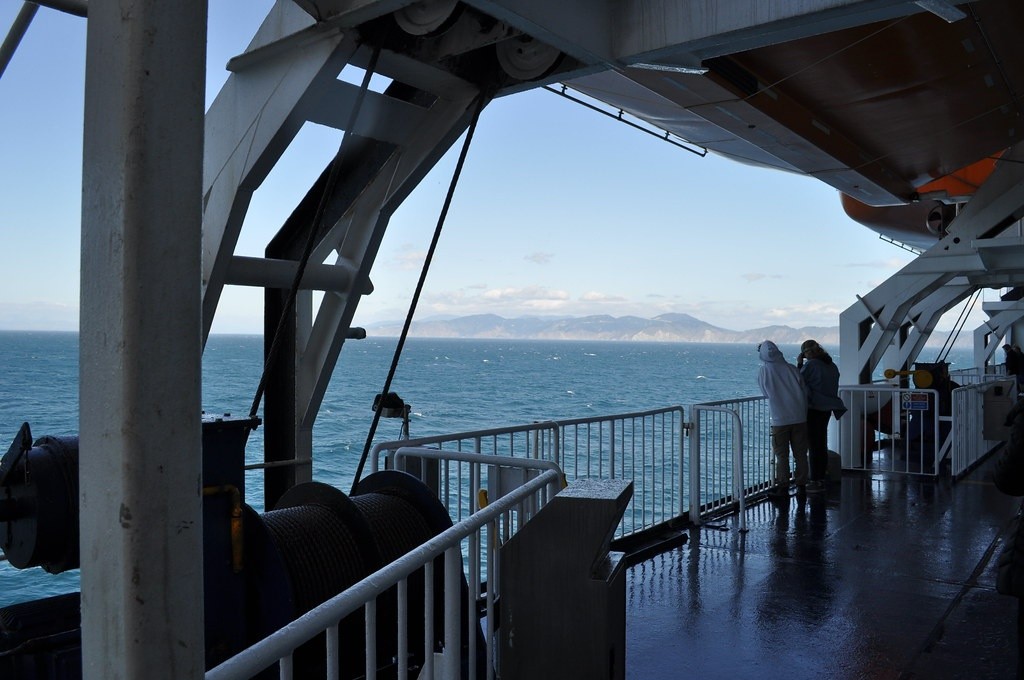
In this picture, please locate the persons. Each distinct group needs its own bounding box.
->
[758,339,810,532]
[1002,344,1024,396]
[797,340,848,494]
